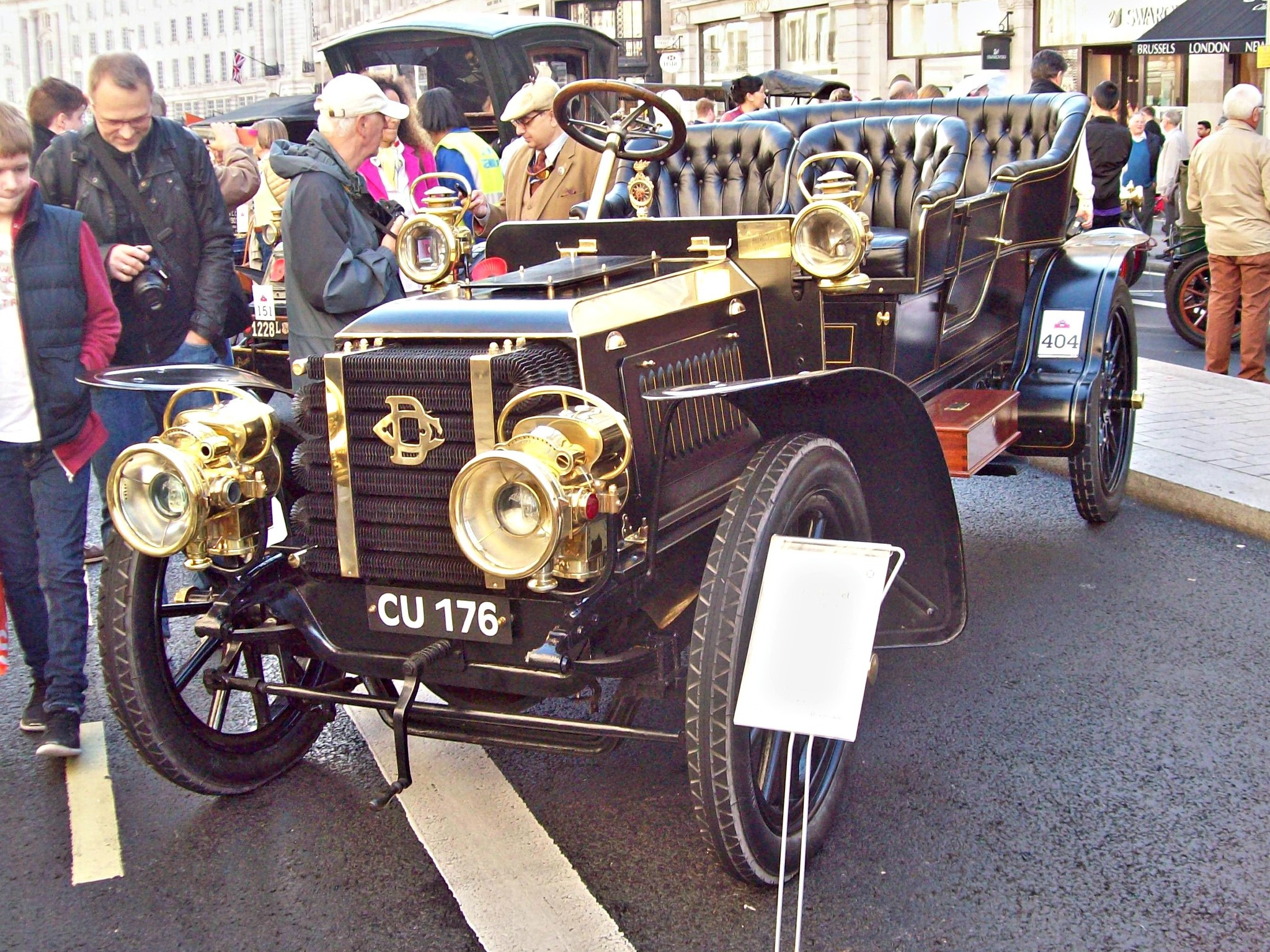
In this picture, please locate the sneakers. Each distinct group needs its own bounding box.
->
[36,710,82,756]
[20,682,47,731]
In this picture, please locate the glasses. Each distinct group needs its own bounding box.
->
[511,111,548,125]
[94,109,152,130]
[1258,106,1266,111]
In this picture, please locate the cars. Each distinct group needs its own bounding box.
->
[91,76,1155,891]
[313,0,670,164]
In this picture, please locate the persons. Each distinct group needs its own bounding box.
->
[653,76,767,126]
[0,98,121,757]
[35,54,239,662]
[27,73,623,563]
[1025,49,1229,262]
[1187,83,1270,384]
[829,79,993,101]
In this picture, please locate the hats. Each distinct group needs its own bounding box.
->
[313,72,409,119]
[500,77,561,122]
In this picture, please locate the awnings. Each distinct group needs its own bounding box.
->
[1132,0,1268,55]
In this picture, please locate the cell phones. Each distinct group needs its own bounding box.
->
[454,184,468,198]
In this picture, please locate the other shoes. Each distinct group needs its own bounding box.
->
[1163,256,1170,261]
[83,544,105,563]
[163,640,171,659]
[1162,226,1167,232]
[1153,214,1158,218]
[1155,252,1169,259]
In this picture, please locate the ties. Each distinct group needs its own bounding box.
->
[531,150,547,197]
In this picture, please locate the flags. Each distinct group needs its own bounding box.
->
[232,51,247,84]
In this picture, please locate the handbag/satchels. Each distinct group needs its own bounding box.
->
[224,270,253,338]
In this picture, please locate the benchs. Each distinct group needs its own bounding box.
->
[729,91,1091,273]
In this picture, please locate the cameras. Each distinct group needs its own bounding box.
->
[377,200,405,226]
[123,256,171,314]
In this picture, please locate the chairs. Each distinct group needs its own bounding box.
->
[567,114,973,297]
